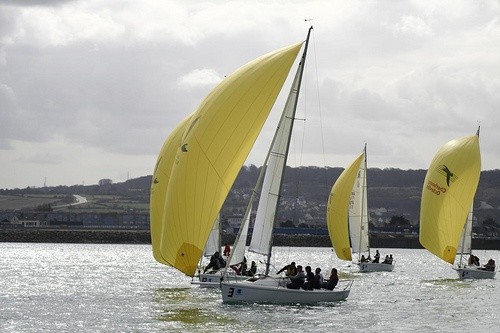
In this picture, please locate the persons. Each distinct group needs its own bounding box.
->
[275,262,340,291]
[205,243,257,277]
[361,250,393,265]
[468,255,495,272]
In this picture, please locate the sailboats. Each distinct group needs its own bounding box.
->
[149,111,265,288]
[159,17,355,305]
[418,119,498,281]
[326,143,397,274]
